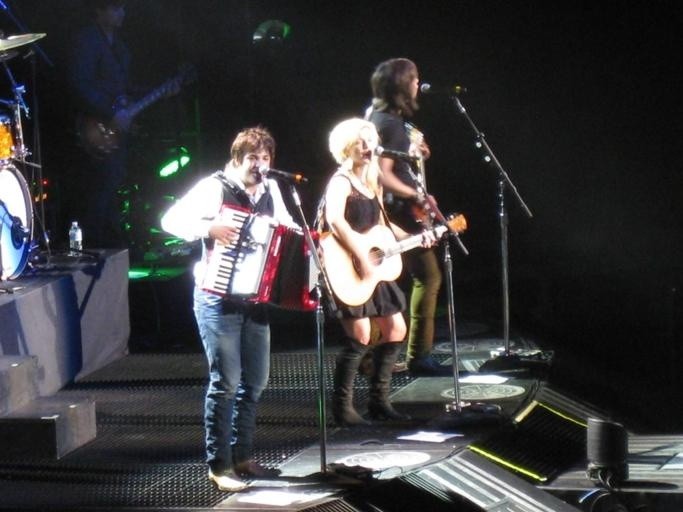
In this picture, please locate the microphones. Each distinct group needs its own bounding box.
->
[420,82,469,96]
[374,146,420,163]
[259,165,308,186]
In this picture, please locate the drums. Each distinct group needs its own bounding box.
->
[0,163,35,282]
[0,115,15,164]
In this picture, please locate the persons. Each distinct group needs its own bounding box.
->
[312,116,439,430]
[353,56,457,379]
[60,0,182,251]
[160,123,324,494]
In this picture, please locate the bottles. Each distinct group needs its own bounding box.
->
[68,220,82,257]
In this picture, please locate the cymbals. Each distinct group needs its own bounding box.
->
[0,30,47,61]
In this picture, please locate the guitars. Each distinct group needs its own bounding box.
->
[320,213,466,307]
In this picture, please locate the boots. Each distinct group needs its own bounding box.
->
[367,342,410,422]
[332,336,367,425]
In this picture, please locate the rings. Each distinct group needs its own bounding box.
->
[224,233,228,238]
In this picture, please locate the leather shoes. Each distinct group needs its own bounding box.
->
[419,360,454,375]
[208,467,248,492]
[393,362,407,371]
[237,459,280,476]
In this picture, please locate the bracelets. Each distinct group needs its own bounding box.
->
[413,189,421,202]
